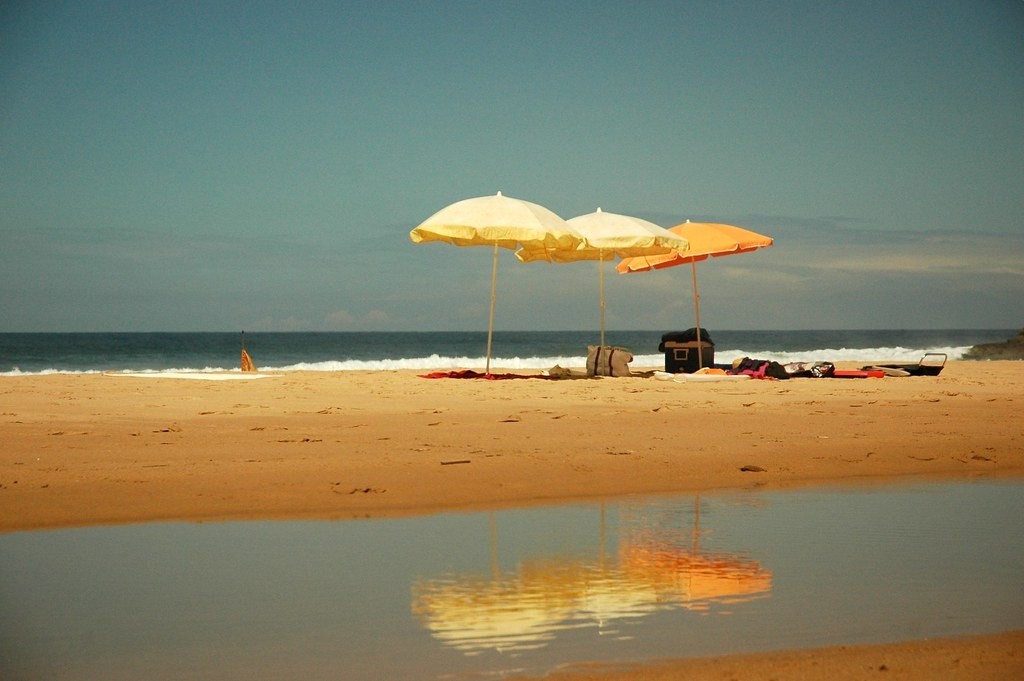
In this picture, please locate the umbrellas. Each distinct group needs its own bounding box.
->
[514,206,691,377]
[616,217,774,371]
[409,192,586,377]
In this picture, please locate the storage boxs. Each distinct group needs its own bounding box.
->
[664,341,715,373]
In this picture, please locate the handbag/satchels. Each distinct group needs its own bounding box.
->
[586,346,633,378]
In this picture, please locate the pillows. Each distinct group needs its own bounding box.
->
[586,344,632,377]
